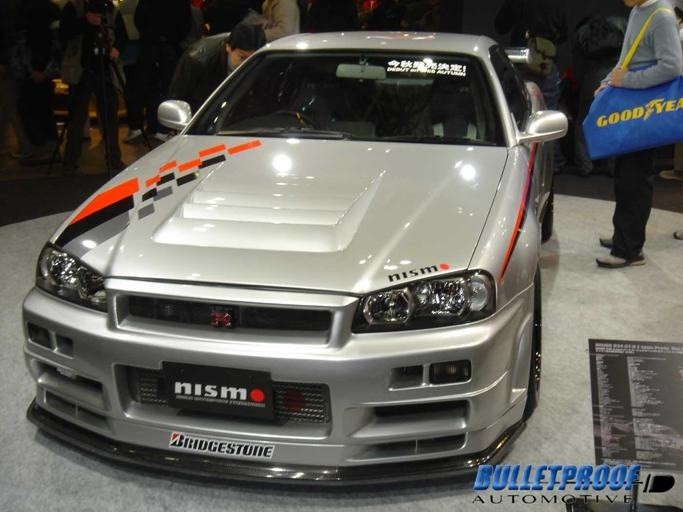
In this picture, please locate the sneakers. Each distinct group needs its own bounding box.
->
[599,236,617,247]
[11,129,173,179]
[596,249,648,269]
[673,229,683,240]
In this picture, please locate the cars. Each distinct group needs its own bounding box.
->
[23,30,569,487]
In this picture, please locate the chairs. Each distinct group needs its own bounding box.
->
[292,74,359,133]
[416,75,481,140]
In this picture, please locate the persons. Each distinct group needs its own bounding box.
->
[0,0,682,182]
[592,0,682,269]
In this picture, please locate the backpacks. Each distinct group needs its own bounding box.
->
[579,16,625,72]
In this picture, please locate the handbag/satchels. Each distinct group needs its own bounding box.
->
[581,6,683,160]
[523,33,558,78]
